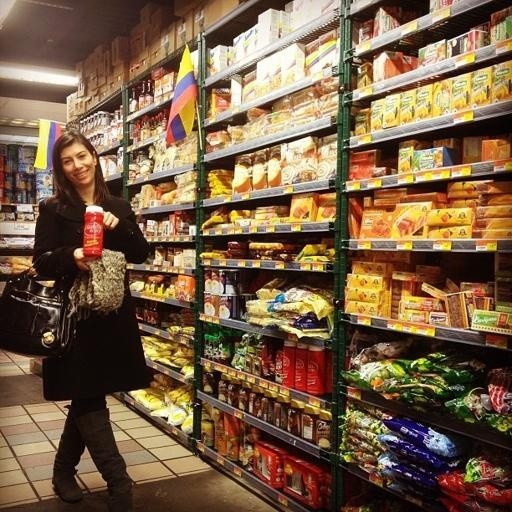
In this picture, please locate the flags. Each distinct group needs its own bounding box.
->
[164,45,197,145]
[32,119,65,171]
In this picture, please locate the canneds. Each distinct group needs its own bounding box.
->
[203,269,256,320]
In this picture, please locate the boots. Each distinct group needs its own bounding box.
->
[50,404,88,502]
[76,407,135,512]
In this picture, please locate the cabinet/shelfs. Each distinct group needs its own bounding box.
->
[339,0,512,512]
[202,2,346,512]
[61,35,200,455]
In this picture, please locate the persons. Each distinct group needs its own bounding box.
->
[31,129,150,512]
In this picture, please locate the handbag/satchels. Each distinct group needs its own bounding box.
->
[0,250,77,358]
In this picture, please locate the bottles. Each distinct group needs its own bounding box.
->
[201,418,215,447]
[81,205,105,257]
[79,78,171,148]
[278,338,335,398]
